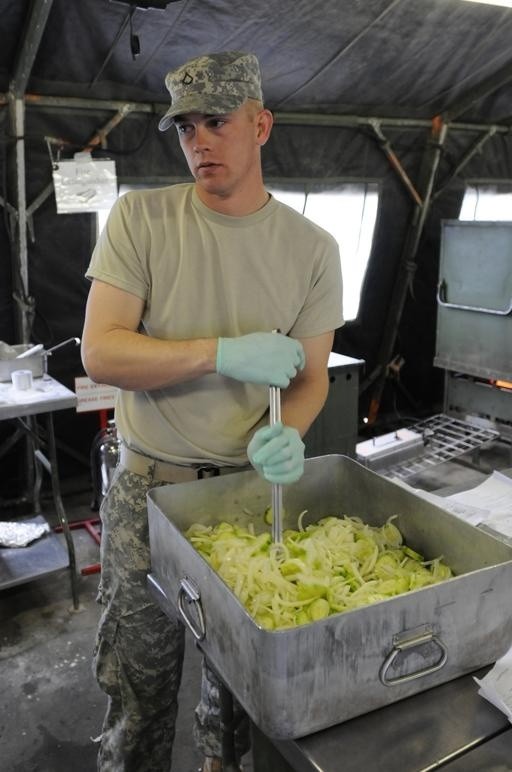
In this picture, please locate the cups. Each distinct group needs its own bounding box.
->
[10,368,34,390]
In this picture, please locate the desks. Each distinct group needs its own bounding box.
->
[147,477,512,772]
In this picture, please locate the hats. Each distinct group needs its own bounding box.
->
[158,50,262,132]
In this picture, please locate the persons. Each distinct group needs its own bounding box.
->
[81,51,346,772]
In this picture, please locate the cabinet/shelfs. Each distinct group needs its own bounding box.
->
[0,375,84,615]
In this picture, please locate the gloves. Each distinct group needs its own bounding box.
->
[246,424,305,483]
[217,332,305,389]
